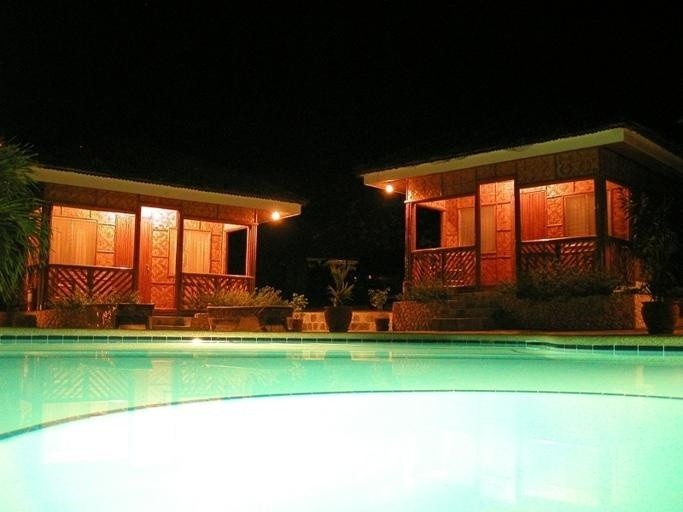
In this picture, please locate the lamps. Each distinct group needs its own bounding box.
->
[384,181,393,193]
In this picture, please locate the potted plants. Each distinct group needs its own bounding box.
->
[367,284,392,331]
[321,256,356,332]
[615,180,680,334]
[285,292,310,332]
